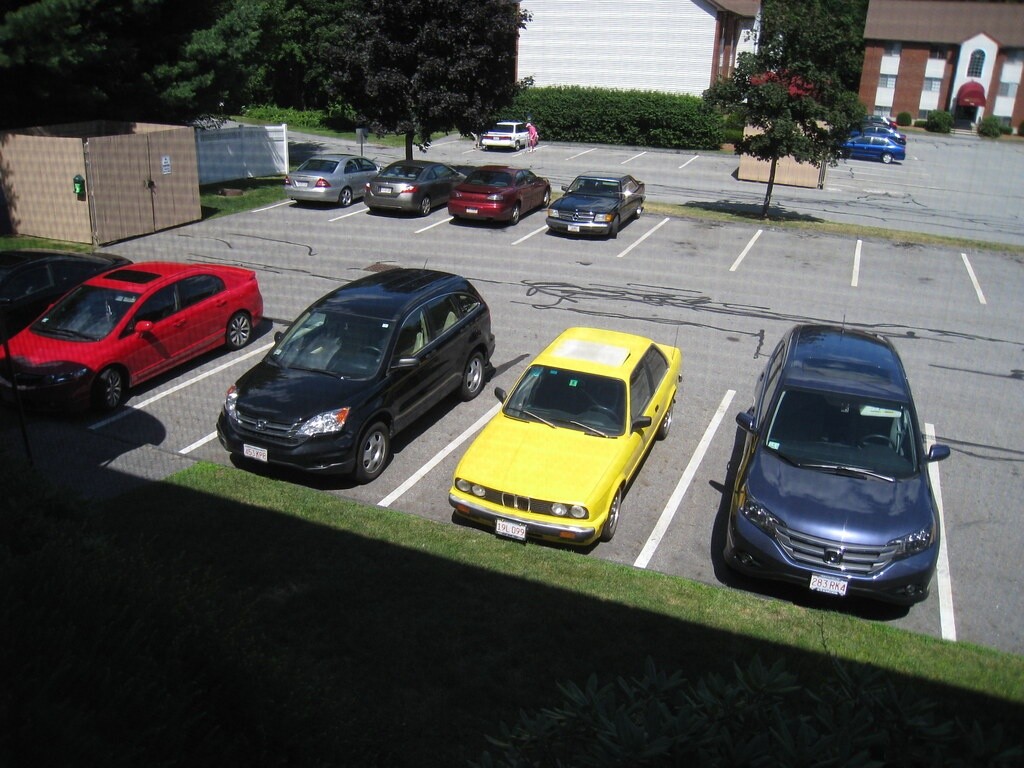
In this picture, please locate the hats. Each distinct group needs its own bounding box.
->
[526,123,532,127]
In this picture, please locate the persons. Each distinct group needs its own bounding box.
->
[471,131,481,150]
[526,123,537,152]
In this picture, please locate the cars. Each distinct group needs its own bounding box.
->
[364,160,468,217]
[0,248,137,344]
[447,164,551,227]
[546,171,646,239]
[836,134,906,164]
[480,122,542,152]
[447,327,683,545]
[284,154,386,208]
[851,125,906,146]
[0,261,264,418]
[860,116,897,130]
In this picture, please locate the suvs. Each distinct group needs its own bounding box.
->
[719,325,950,606]
[215,267,496,484]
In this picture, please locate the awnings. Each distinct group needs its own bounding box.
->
[958,81,986,107]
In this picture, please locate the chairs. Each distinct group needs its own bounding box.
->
[494,182,508,186]
[394,314,423,356]
[326,314,369,353]
[471,180,484,184]
[589,382,619,418]
